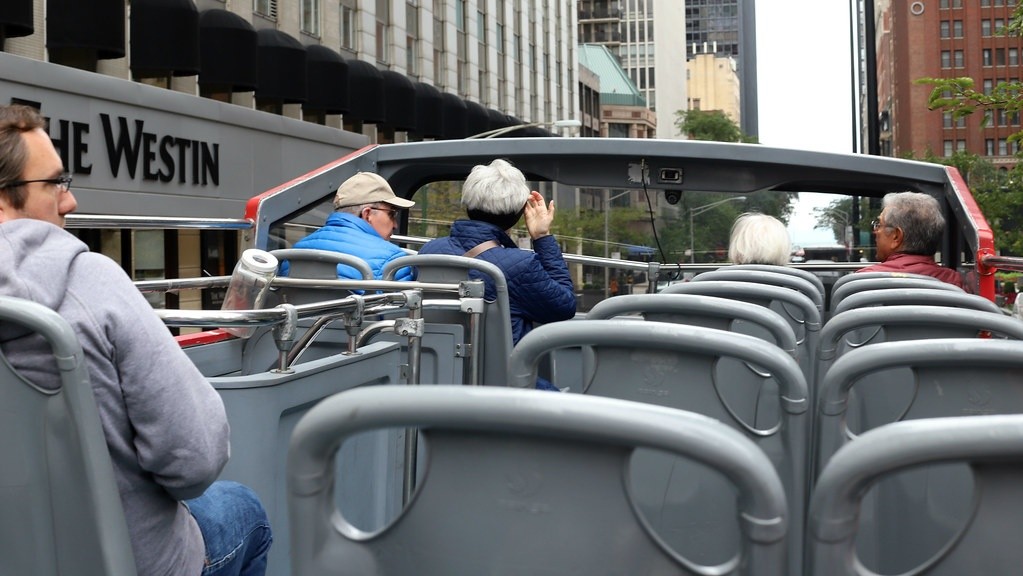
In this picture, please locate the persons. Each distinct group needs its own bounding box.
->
[850,190,971,294]
[0,102,273,576]
[727,209,792,268]
[412,157,577,393]
[274,170,417,290]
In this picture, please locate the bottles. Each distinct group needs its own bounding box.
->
[218,249,278,339]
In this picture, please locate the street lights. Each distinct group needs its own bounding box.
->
[809,205,851,246]
[688,196,747,263]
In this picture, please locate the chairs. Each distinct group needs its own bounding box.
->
[2,243,1022,576]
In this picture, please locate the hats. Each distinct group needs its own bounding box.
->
[330,170,416,213]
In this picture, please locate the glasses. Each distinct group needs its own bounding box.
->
[0,175,73,194]
[870,218,898,231]
[368,205,399,218]
